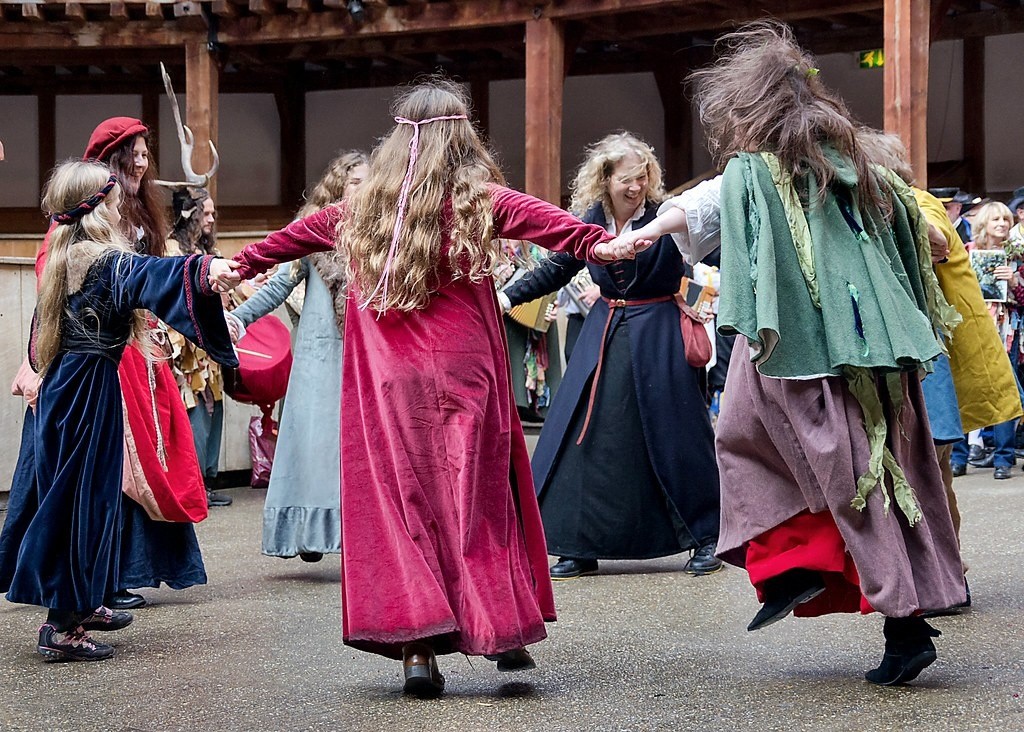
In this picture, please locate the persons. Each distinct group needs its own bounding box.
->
[607,17,969,685]
[161,152,369,563]
[0,116,241,662]
[210,85,652,696]
[494,239,737,432]
[496,131,723,581]
[860,129,1024,618]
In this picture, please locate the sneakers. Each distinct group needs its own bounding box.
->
[80,605,132,633]
[36,625,117,663]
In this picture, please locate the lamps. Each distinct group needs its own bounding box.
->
[348,0,363,16]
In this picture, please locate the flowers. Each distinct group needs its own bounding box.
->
[1000,235,1024,258]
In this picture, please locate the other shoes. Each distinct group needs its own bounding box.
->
[403,644,443,696]
[300,550,321,562]
[206,487,233,507]
[747,565,826,632]
[483,646,535,672]
[865,617,939,686]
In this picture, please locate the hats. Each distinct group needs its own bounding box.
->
[1006,188,1024,216]
[928,186,970,205]
[84,116,148,164]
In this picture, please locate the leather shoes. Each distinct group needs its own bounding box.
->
[969,443,985,461]
[969,446,1016,467]
[949,462,965,478]
[993,466,1010,479]
[690,541,721,575]
[550,553,599,578]
[1014,450,1024,458]
[103,589,145,609]
